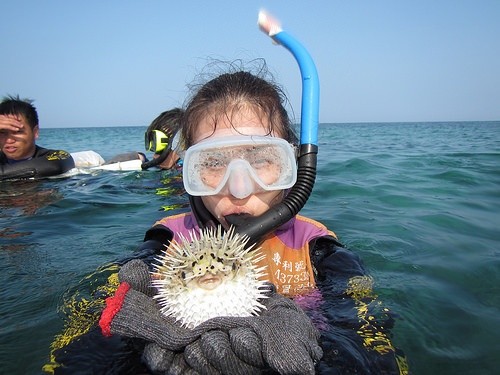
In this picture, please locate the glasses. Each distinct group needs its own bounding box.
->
[182,136,299,199]
[144,130,169,153]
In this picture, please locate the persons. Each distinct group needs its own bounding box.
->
[0,95,75,182]
[100,72,409,375]
[145,109,187,171]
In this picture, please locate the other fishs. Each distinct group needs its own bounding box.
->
[149,223,272,331]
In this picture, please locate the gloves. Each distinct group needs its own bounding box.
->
[98,259,323,375]
